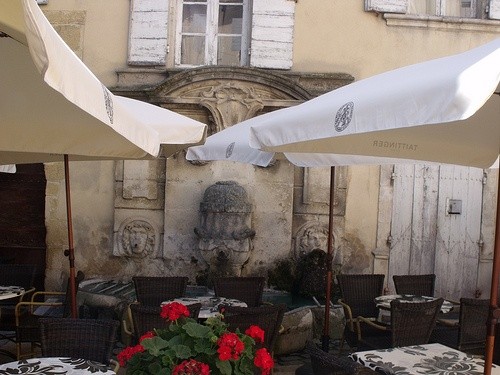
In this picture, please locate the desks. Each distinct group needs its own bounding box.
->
[0,357,116,375]
[351,343,500,375]
[375,295,453,326]
[0,285,35,359]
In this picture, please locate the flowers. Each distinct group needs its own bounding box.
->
[117,303,272,375]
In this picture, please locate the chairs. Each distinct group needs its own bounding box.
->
[336,273,385,347]
[393,274,436,296]
[212,276,265,304]
[38,318,120,373]
[220,303,286,360]
[132,276,189,307]
[435,296,491,351]
[309,342,360,375]
[355,298,443,349]
[123,304,201,345]
[15,270,85,361]
